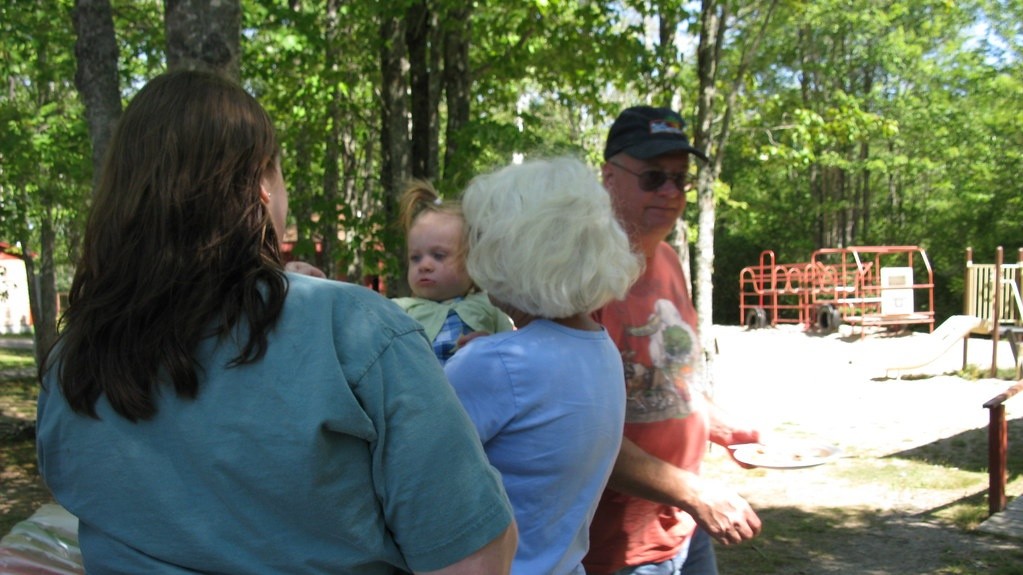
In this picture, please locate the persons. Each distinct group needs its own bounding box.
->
[36,71,519,575]
[380,153,626,575]
[583,105,761,574]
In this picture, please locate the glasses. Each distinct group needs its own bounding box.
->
[610,161,698,193]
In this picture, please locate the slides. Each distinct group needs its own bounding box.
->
[877,315,981,370]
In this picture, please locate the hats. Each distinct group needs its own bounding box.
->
[604,105,710,163]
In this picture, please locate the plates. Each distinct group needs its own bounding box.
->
[733,442,834,470]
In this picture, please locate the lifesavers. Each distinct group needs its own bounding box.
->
[746,306,766,329]
[817,303,838,334]
